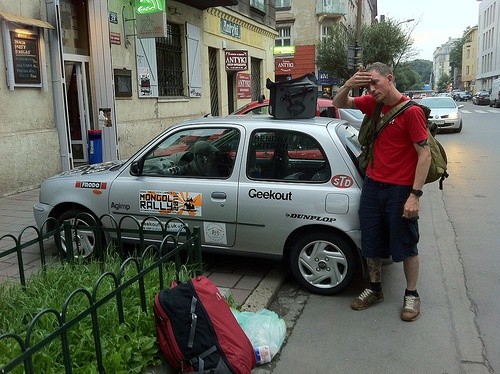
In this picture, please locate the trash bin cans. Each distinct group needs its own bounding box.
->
[88,130,102,164]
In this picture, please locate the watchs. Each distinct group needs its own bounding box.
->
[411,189,423,196]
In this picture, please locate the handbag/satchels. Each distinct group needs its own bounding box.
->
[357,100,449,190]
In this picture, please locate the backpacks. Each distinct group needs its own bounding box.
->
[153,275,257,374]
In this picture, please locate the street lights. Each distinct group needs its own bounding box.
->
[389,19,416,69]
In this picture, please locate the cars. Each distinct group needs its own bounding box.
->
[405,88,500,109]
[33,115,394,296]
[148,93,365,161]
[417,96,465,133]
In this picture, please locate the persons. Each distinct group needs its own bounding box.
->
[332,61,431,321]
[409,89,413,99]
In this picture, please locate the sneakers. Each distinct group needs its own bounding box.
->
[351,287,384,310]
[401,293,420,321]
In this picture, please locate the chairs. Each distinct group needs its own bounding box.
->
[248,148,262,178]
